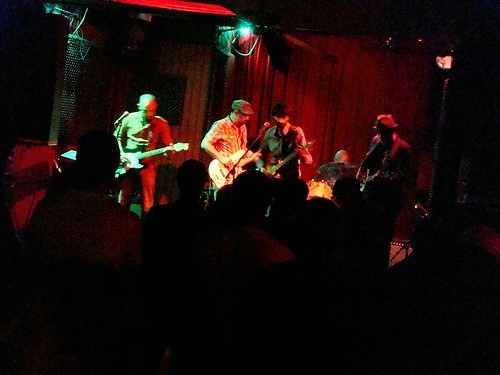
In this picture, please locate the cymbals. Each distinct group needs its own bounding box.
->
[316,161,356,178]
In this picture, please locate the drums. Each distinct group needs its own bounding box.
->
[305,178,339,212]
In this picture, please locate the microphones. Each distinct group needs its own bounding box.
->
[114,110,129,125]
[263,122,270,126]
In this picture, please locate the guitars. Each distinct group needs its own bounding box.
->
[262,140,317,179]
[114,142,190,180]
[359,168,403,193]
[207,143,263,190]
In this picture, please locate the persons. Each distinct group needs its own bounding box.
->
[0,100,500,375]
[113,94,174,211]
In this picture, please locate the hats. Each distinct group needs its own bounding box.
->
[377,114,397,129]
[232,100,253,115]
